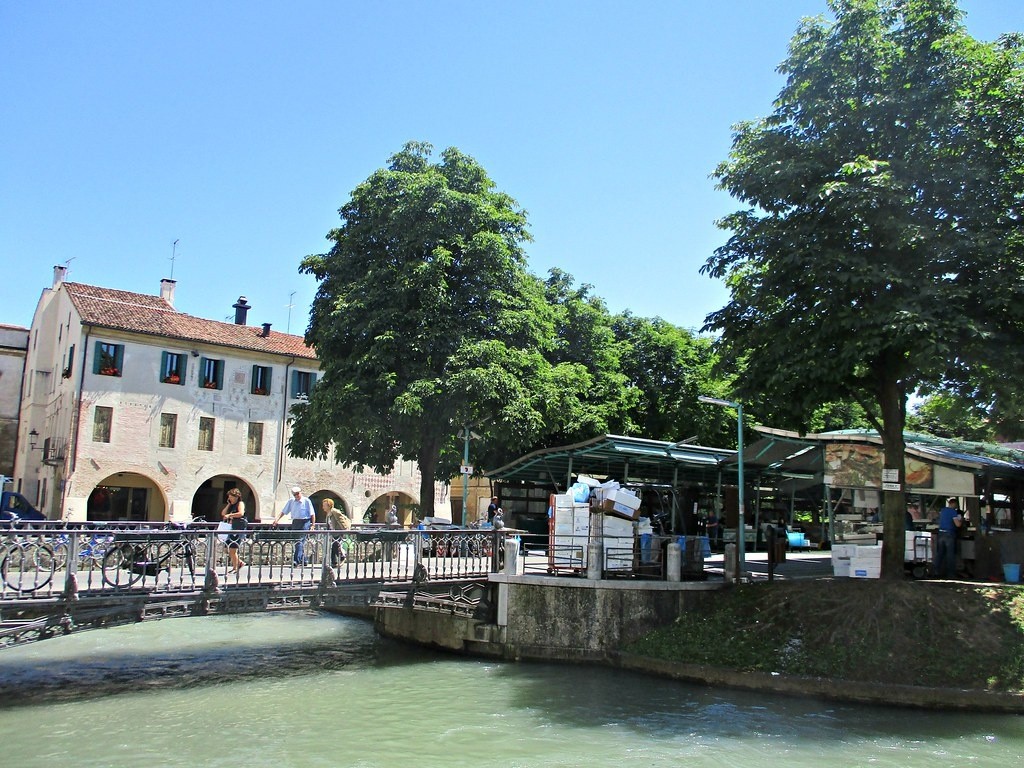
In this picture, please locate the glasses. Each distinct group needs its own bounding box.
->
[294,492,299,496]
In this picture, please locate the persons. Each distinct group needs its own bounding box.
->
[322,498,348,568]
[757,517,805,552]
[935,499,963,578]
[272,487,316,566]
[487,496,498,525]
[222,488,248,574]
[705,510,727,550]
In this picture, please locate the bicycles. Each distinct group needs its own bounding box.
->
[0,513,206,593]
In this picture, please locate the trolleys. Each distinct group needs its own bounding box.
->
[904,535,932,578]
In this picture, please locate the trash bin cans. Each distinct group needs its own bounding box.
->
[774,527,786,564]
[418,516,461,558]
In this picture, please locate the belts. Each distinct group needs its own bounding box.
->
[939,529,946,532]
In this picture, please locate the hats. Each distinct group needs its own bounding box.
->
[291,487,301,493]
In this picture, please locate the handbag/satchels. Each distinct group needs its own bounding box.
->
[217,517,232,542]
[342,535,354,550]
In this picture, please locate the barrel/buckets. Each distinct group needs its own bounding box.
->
[1003,564,1020,584]
[641,533,652,562]
[677,535,686,563]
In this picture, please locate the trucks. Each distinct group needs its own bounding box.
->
[0,474,48,537]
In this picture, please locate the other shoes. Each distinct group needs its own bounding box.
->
[293,562,307,567]
[228,569,237,574]
[239,561,246,568]
[331,556,346,568]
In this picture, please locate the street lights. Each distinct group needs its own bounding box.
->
[698,395,746,577]
